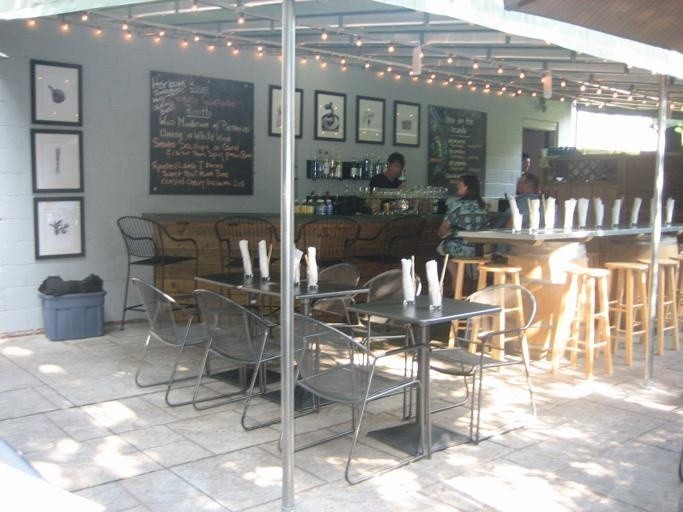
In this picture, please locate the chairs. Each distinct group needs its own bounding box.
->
[116,216,202,329]
[317,263,361,364]
[212,209,438,378]
[190,286,316,432]
[128,277,244,408]
[404,284,537,444]
[278,311,424,487]
[363,268,420,375]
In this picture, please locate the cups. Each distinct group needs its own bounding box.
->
[512,210,675,232]
[425,284,445,311]
[294,263,300,285]
[305,266,319,288]
[402,283,416,305]
[260,263,269,281]
[243,271,254,289]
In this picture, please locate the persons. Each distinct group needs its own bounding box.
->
[495,174,541,255]
[436,174,490,259]
[521,152,534,173]
[368,152,406,189]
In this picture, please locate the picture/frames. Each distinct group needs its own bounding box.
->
[33,195,87,261]
[267,84,303,139]
[28,129,86,194]
[27,58,85,126]
[355,94,384,143]
[313,89,346,141]
[391,100,420,147]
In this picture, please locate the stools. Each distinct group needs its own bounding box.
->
[448,258,491,354]
[545,267,614,379]
[465,263,530,373]
[594,261,650,366]
[631,247,681,357]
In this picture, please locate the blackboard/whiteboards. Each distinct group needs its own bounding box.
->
[149,72,253,194]
[427,104,487,197]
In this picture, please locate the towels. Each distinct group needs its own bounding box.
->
[508,193,675,233]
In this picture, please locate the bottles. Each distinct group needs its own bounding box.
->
[312,150,406,180]
[296,186,449,215]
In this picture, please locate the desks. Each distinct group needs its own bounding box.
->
[319,282,365,407]
[346,294,501,456]
